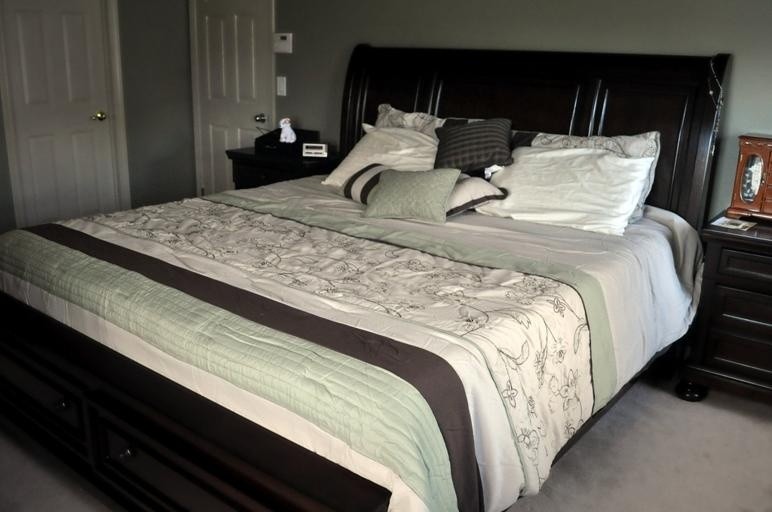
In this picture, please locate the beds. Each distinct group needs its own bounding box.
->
[0,42,734,512]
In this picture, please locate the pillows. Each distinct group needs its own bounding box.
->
[321,103,661,237]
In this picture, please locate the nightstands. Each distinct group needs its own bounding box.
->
[674,212,771,403]
[224,145,343,190]
